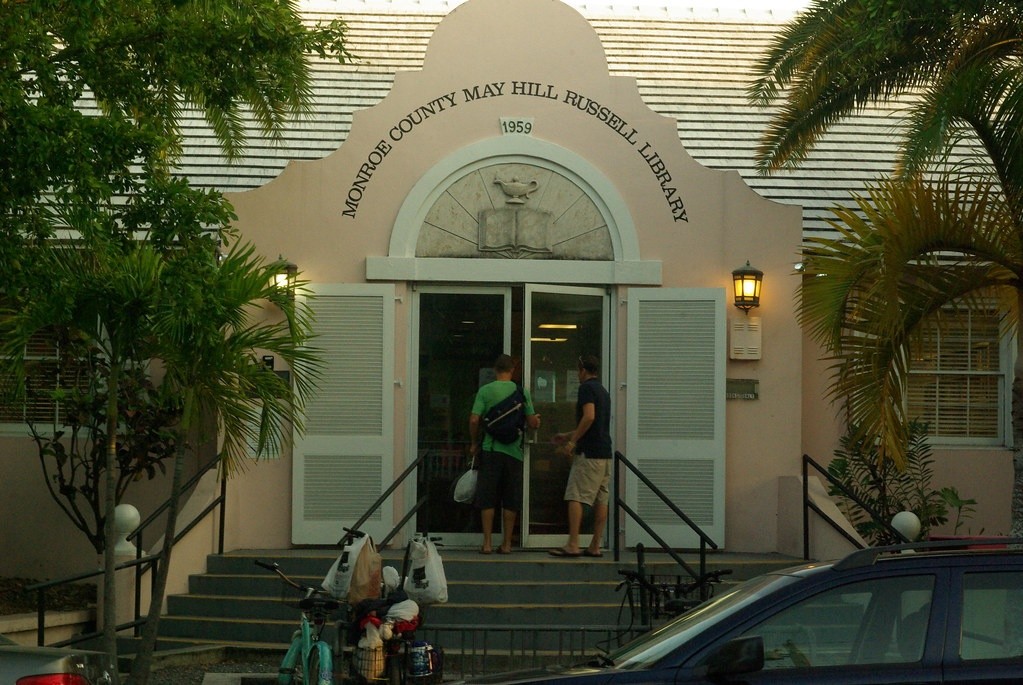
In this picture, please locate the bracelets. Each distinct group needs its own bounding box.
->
[568,441,576,448]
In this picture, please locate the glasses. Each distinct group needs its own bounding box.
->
[579,355,583,365]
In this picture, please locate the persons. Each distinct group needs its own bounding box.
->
[469,355,541,553]
[549,355,614,557]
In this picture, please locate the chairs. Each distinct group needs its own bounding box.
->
[897,600,928,658]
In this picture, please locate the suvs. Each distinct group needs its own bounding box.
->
[448,535,1023,685]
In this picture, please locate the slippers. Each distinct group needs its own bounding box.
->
[479,546,492,554]
[496,545,511,554]
[583,549,603,557]
[548,547,583,557]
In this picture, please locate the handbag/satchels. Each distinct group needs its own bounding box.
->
[482,385,525,445]
[453,456,480,505]
[322,533,383,603]
[404,534,447,604]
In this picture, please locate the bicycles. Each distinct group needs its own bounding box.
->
[252,557,348,685]
[341,526,445,685]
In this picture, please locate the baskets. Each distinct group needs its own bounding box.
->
[280,578,318,610]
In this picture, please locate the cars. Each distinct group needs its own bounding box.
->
[0,634,116,685]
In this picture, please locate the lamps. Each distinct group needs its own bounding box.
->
[732,259,764,316]
[264,254,298,308]
